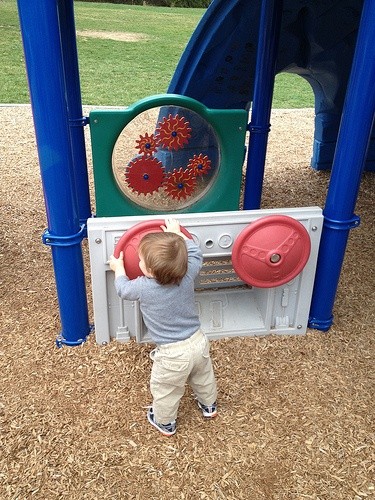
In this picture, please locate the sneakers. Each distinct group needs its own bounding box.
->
[198,400,217,418]
[147,407,177,437]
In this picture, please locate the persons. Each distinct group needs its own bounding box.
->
[109,217,218,436]
